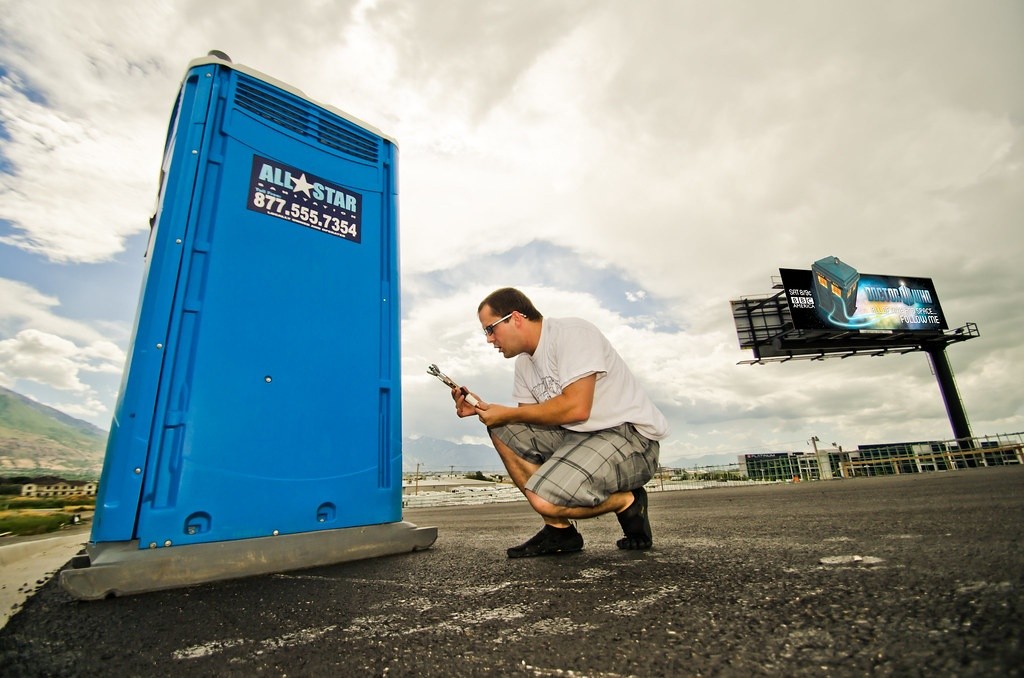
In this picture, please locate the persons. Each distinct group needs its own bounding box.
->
[451,288,668,558]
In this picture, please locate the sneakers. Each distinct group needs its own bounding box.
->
[507,528,584,558]
[616,487,652,548]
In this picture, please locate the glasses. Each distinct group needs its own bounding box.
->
[484,313,526,336]
